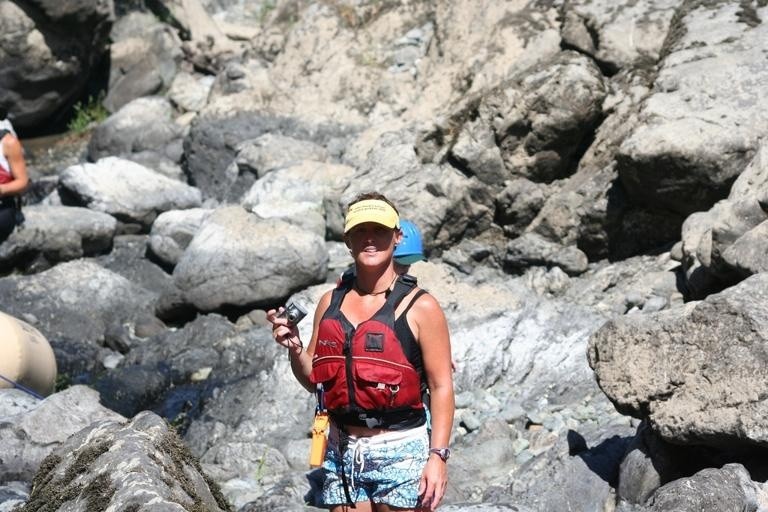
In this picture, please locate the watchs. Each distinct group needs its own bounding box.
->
[429,448,451,461]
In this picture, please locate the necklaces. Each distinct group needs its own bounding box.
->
[353,272,398,299]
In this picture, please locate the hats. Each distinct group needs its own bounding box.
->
[343,197,399,235]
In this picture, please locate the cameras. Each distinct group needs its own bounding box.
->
[277,301,308,326]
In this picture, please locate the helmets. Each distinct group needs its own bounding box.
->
[392,220,429,266]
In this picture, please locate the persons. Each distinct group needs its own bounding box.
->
[0,105,30,249]
[266,190,456,511]
[338,219,426,287]
